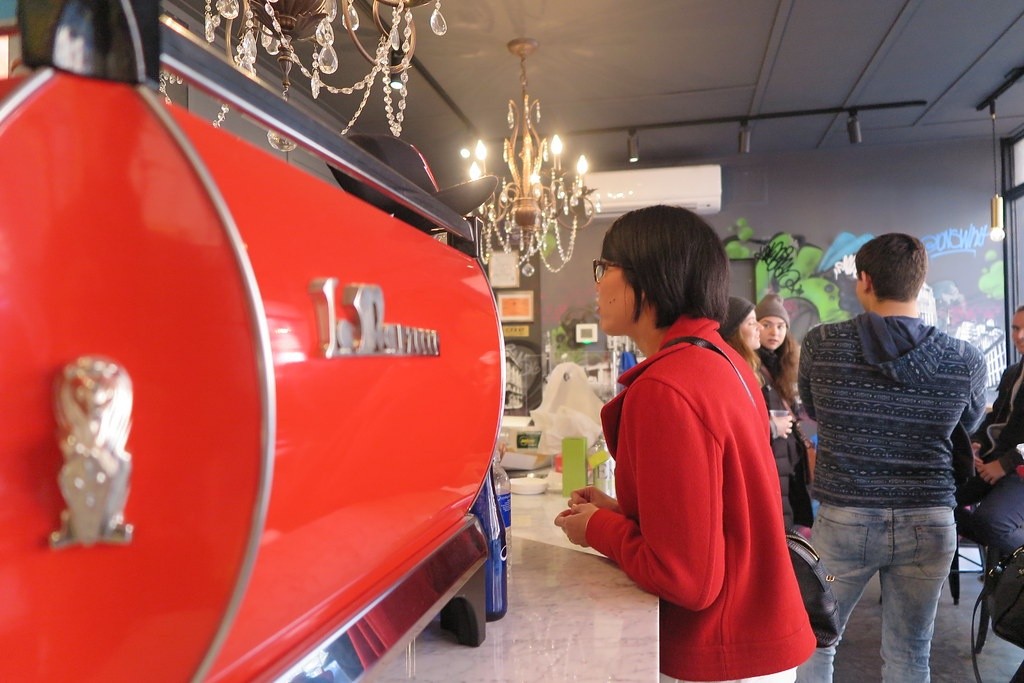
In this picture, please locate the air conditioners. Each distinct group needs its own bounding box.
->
[581,163,722,221]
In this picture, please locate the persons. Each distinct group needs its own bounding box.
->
[794,233,988,683]
[754,293,801,404]
[554,206,818,683]
[717,296,815,533]
[970,306,1024,552]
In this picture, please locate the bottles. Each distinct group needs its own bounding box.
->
[493,457,512,577]
[469,464,507,621]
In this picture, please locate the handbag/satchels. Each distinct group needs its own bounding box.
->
[783,528,839,648]
[989,544,1024,650]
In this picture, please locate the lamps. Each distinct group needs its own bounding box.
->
[456,36,604,274]
[846,111,862,144]
[738,121,752,154]
[625,126,641,162]
[989,101,1004,241]
[153,0,449,151]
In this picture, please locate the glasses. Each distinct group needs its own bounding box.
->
[593,259,636,283]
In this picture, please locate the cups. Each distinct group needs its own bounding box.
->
[770,410,789,418]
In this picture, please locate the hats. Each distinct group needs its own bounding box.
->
[755,294,790,330]
[717,296,755,340]
